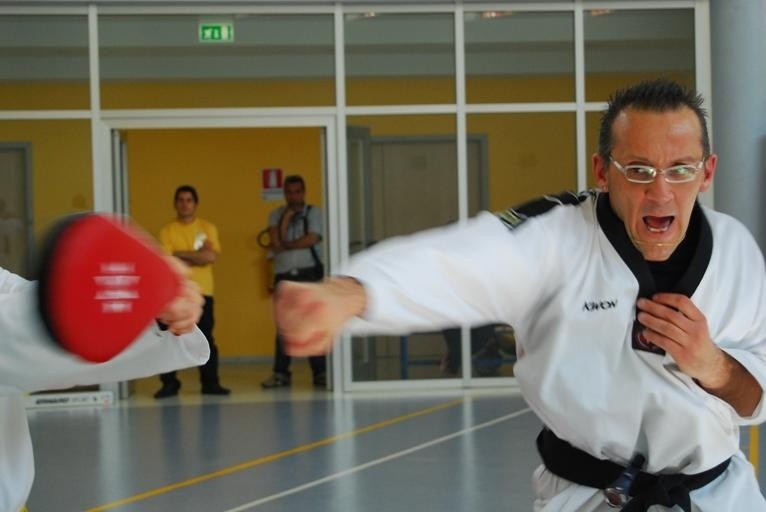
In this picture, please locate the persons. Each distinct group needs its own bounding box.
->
[154,183,232,399]
[0,257,210,512]
[261,173,332,391]
[274,75,766,512]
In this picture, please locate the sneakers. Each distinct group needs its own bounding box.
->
[202,384,230,395]
[313,376,326,386]
[154,388,177,398]
[262,374,289,387]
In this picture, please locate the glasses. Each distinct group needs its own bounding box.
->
[606,154,705,184]
